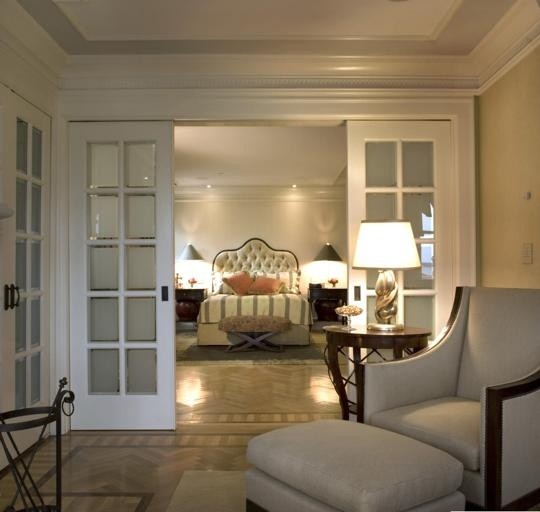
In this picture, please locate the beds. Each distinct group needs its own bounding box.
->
[197,238,311,347]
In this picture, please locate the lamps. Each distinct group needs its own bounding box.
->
[312,243,343,287]
[179,244,203,288]
[351,218,423,333]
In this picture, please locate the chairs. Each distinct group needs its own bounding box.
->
[362,288,540,511]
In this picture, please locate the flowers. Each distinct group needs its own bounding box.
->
[328,278,338,284]
[335,305,362,317]
[188,277,196,284]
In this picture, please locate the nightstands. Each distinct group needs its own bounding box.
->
[176,289,206,322]
[309,288,347,326]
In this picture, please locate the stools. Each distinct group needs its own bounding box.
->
[243,418,468,511]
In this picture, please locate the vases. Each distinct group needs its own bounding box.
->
[333,283,335,288]
[347,316,352,332]
[191,282,193,288]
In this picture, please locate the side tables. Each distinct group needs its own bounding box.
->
[321,321,431,423]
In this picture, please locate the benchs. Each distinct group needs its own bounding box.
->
[219,315,291,353]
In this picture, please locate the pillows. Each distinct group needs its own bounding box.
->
[248,276,283,295]
[210,271,237,296]
[222,270,253,296]
